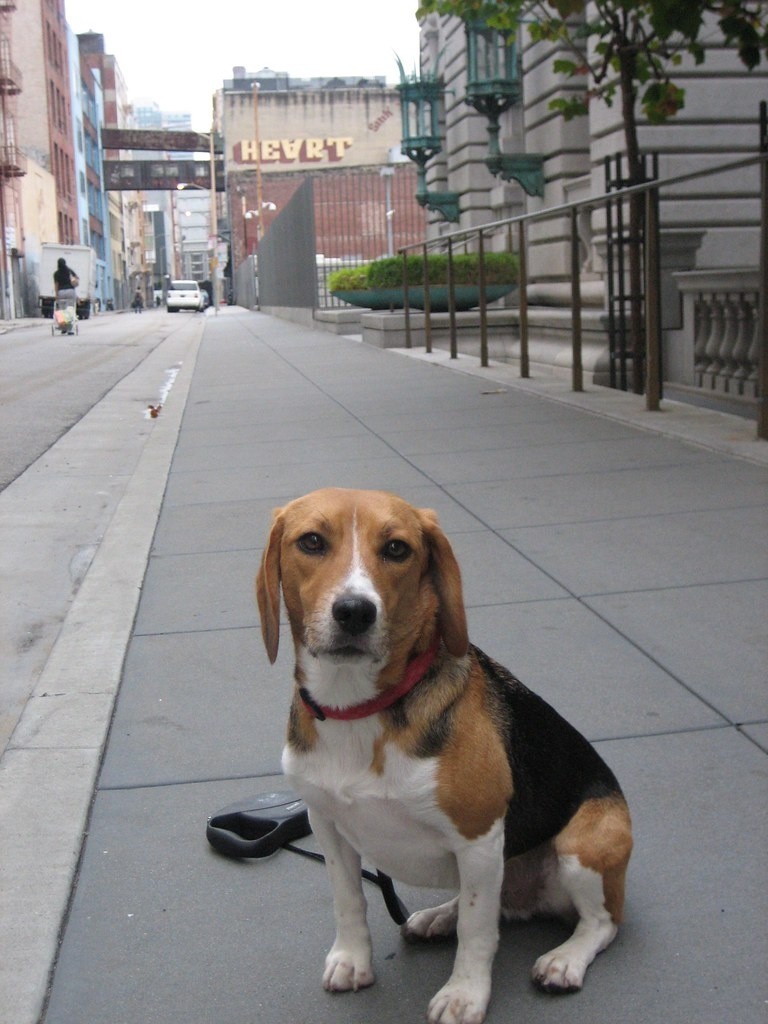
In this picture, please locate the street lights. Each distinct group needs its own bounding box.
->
[176,182,222,309]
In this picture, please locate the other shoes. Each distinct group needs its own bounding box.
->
[62,330,67,333]
[68,332,74,335]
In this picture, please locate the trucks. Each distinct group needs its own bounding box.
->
[37,243,103,320]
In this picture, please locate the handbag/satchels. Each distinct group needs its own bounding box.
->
[69,271,78,287]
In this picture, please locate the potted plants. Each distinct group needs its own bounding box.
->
[370,251,518,310]
[328,266,372,308]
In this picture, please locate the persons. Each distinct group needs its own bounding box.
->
[134,286,143,313]
[53,257,79,335]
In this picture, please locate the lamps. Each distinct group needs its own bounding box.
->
[395,81,460,224]
[460,6,546,198]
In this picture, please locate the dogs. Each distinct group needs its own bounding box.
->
[255,487,635,1024]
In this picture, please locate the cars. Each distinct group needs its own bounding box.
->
[164,280,211,313]
